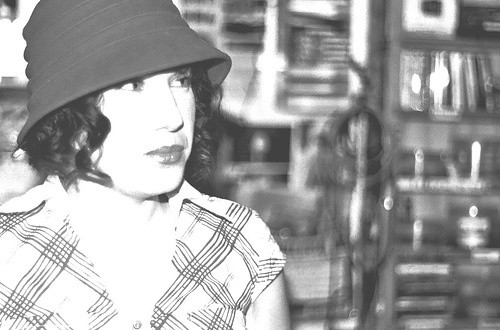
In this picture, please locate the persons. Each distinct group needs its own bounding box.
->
[0,0,298,329]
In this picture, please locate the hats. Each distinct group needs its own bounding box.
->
[17,0,233,152]
